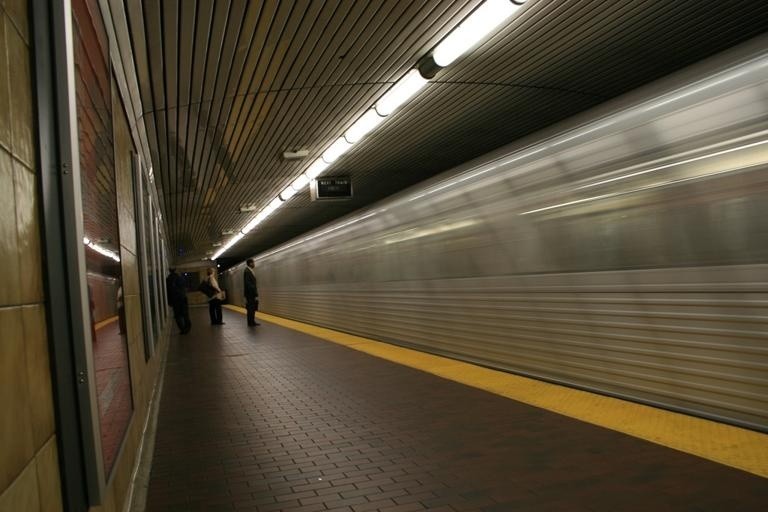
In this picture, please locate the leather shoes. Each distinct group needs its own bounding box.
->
[179,326,191,334]
[211,322,225,325]
[248,323,260,327]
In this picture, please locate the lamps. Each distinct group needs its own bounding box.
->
[210,0,525,263]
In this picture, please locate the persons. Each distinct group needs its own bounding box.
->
[88,280,98,344]
[244,258,261,327]
[114,275,127,336]
[206,267,225,325]
[164,264,193,335]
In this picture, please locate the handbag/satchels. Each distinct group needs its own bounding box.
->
[246,300,258,311]
[198,281,216,298]
